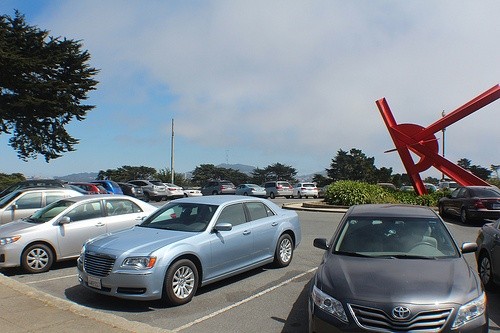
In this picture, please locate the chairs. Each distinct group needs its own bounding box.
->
[407,224,438,252]
[75,203,95,220]
[192,206,211,226]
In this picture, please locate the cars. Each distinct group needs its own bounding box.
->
[235,184,266,199]
[0,193,176,273]
[475,218,500,292]
[424,183,436,192]
[289,183,319,199]
[399,186,415,192]
[308,203,489,333]
[183,189,204,198]
[0,189,113,226]
[0,178,108,209]
[117,181,144,200]
[162,183,185,201]
[89,179,123,195]
[438,186,500,224]
[77,194,301,307]
[318,184,329,198]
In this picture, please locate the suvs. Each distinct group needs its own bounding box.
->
[377,183,395,192]
[128,178,167,202]
[201,180,237,196]
[260,181,294,199]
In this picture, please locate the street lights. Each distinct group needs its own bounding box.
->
[442,109,447,182]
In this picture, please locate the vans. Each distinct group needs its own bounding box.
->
[441,181,458,193]
